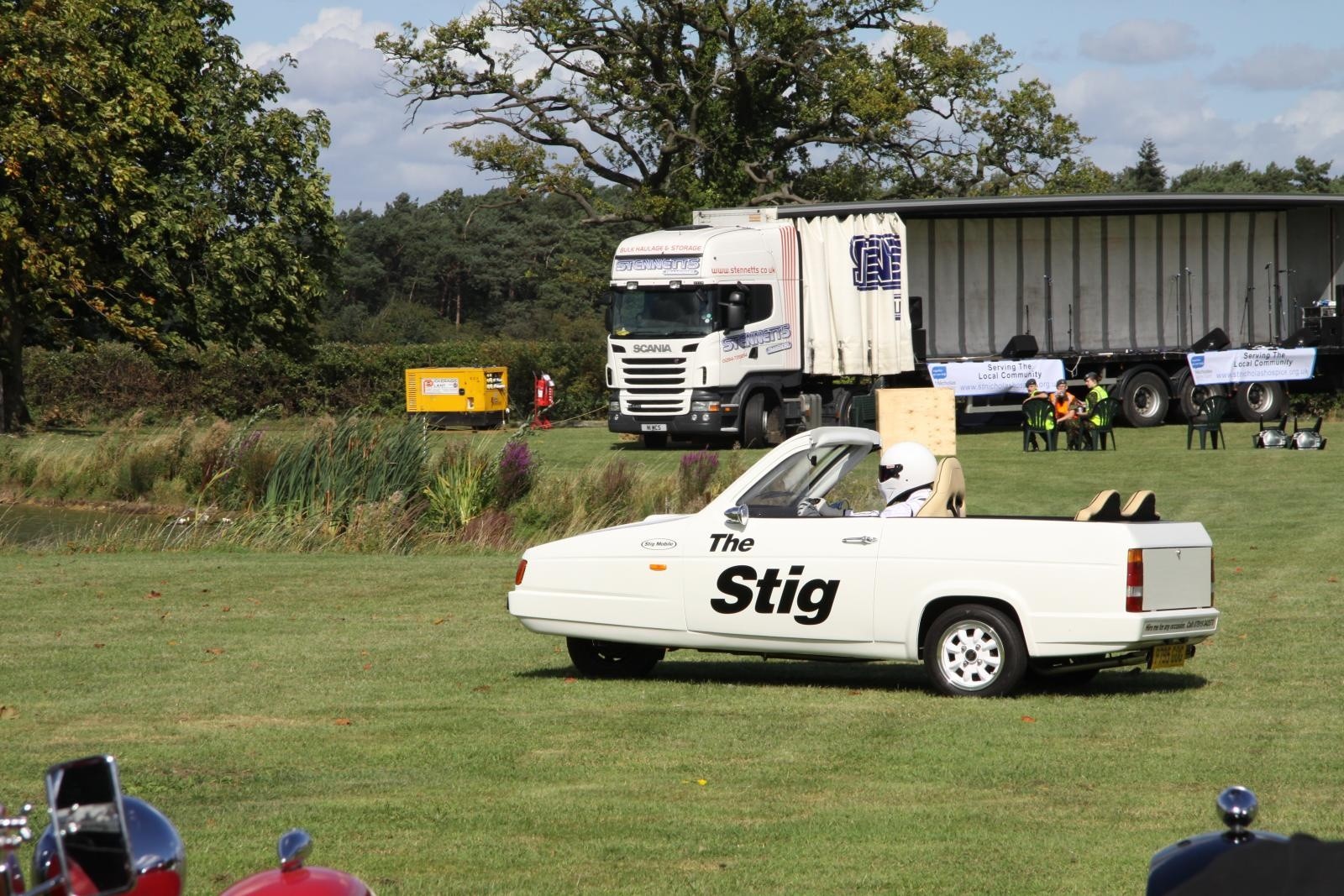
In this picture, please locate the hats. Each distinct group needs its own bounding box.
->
[1057,379,1067,386]
[1025,379,1036,387]
[1084,372,1096,381]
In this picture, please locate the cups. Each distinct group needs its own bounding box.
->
[1079,407,1082,413]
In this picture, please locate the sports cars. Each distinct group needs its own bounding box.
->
[505,423,1223,700]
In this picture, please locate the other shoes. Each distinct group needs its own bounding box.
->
[1046,445,1049,450]
[1033,445,1039,450]
[1067,443,1093,450]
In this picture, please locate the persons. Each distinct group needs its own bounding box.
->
[1022,379,1056,452]
[1066,372,1109,451]
[1048,379,1077,451]
[796,442,939,517]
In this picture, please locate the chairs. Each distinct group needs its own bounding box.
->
[1251,413,1292,449]
[914,457,965,517]
[1076,397,1122,451]
[1294,415,1328,449]
[1186,395,1227,451]
[1022,398,1056,453]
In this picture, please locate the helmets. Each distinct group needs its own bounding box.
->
[878,442,938,507]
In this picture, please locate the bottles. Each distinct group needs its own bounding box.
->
[1060,390,1065,397]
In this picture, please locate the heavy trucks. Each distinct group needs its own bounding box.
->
[602,206,1344,451]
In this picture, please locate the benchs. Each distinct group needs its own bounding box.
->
[1071,488,1160,524]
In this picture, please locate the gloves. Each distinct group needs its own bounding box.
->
[812,497,846,517]
[797,501,821,517]
